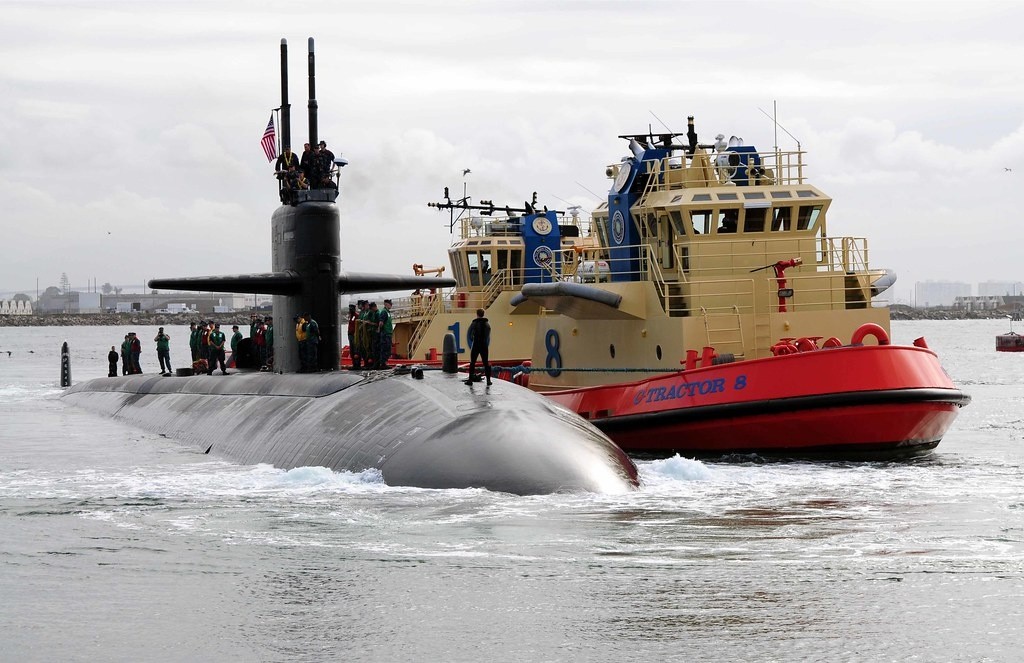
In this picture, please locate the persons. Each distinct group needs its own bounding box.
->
[231,326,242,360]
[250,313,273,371]
[190,321,230,375]
[293,312,322,373]
[108,346,118,377]
[121,333,143,375]
[347,300,392,370]
[275,141,336,190]
[464,308,492,386]
[154,327,172,374]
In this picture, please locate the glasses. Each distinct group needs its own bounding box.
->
[251,316,257,317]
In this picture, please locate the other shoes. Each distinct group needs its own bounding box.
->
[207,372,212,375]
[222,372,230,375]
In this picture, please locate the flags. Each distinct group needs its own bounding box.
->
[261,113,276,163]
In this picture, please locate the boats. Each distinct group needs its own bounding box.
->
[192,116,973,460]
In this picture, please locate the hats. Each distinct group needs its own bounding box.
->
[232,326,239,329]
[384,300,393,304]
[362,300,368,306]
[369,302,376,309]
[356,300,364,305]
